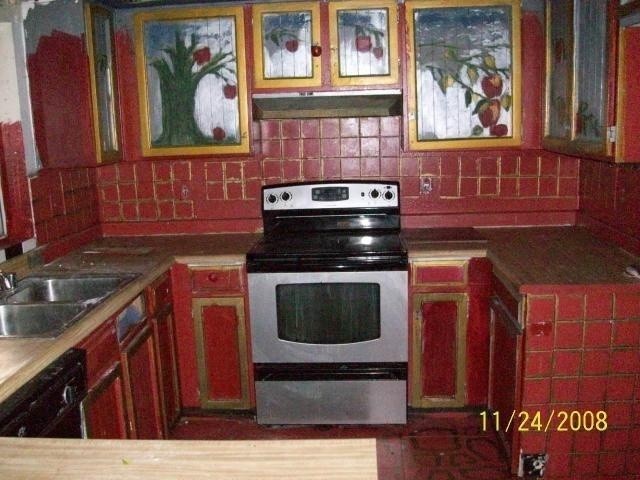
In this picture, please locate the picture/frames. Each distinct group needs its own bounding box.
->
[84,4,122,164]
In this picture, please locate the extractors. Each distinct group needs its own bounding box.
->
[251,90,401,120]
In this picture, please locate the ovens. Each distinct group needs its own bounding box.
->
[246,181,410,423]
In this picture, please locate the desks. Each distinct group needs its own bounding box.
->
[0,438,378,479]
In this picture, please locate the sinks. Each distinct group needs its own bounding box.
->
[0,275,142,339]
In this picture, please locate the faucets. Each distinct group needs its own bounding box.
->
[1,270,11,290]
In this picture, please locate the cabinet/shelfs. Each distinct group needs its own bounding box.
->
[145,271,182,440]
[249,0,403,95]
[173,263,255,412]
[403,2,545,151]
[76,291,164,439]
[541,0,640,164]
[111,5,260,159]
[475,226,640,479]
[410,258,492,410]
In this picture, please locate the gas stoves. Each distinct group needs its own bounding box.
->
[245,227,407,259]
